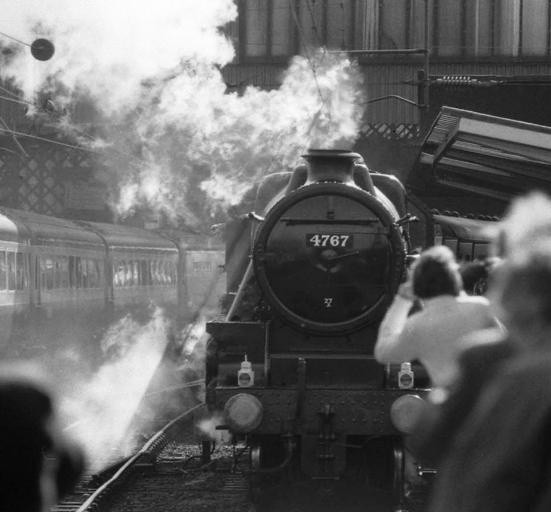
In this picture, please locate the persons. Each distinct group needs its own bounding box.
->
[374,245,501,496]
[415,190,551,512]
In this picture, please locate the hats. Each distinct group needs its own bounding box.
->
[413,245,464,297]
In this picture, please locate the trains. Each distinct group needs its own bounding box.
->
[0,205,228,368]
[204,147,435,512]
